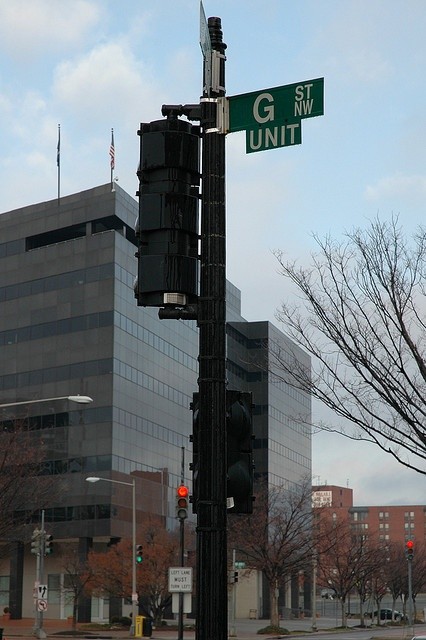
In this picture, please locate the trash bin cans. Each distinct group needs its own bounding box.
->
[142,618,152,637]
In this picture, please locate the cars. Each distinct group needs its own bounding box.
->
[370,608,403,620]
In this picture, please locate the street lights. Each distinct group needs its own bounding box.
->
[85,477,138,636]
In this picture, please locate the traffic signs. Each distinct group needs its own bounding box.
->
[225,76,323,153]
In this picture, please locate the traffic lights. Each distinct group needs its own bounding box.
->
[44,535,53,556]
[407,541,414,560]
[176,486,188,518]
[135,545,142,563]
